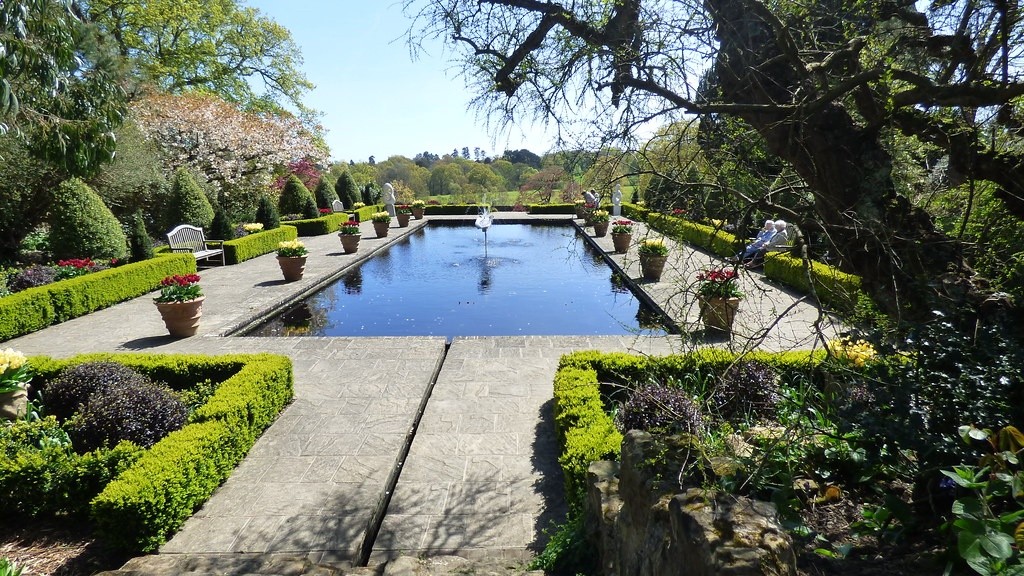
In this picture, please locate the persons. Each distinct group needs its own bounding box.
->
[581,189,600,209]
[724,220,787,267]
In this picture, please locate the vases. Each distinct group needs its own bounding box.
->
[612,233,632,253]
[339,232,361,253]
[154,293,206,337]
[699,296,740,338]
[410,199,426,209]
[372,222,390,237]
[397,213,412,227]
[593,222,609,237]
[640,256,667,279]
[583,214,595,226]
[277,254,308,281]
[411,207,425,219]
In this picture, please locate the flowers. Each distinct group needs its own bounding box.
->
[338,220,360,234]
[696,269,744,299]
[612,220,636,233]
[152,274,202,303]
[395,204,411,214]
[638,238,671,257]
[584,201,597,212]
[592,210,610,223]
[277,238,310,257]
[371,210,391,224]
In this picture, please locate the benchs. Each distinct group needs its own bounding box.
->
[332,200,355,223]
[166,224,225,267]
[750,224,804,269]
